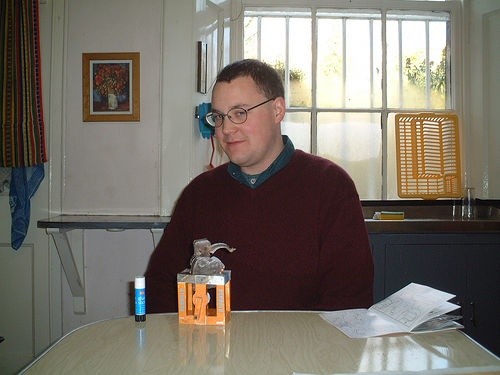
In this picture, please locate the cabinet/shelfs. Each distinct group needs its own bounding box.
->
[362,207,500,357]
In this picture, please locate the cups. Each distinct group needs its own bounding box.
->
[462,188,477,218]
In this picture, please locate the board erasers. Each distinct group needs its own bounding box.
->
[380,211,405,220]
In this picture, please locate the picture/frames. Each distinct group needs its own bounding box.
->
[197,41,208,94]
[82,51,140,122]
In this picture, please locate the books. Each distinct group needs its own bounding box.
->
[319,282,464,338]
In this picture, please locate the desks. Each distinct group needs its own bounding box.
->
[37,214,171,313]
[17,310,500,375]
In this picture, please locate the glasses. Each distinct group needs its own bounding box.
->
[206,98,274,127]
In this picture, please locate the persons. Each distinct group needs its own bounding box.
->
[143,59,374,315]
